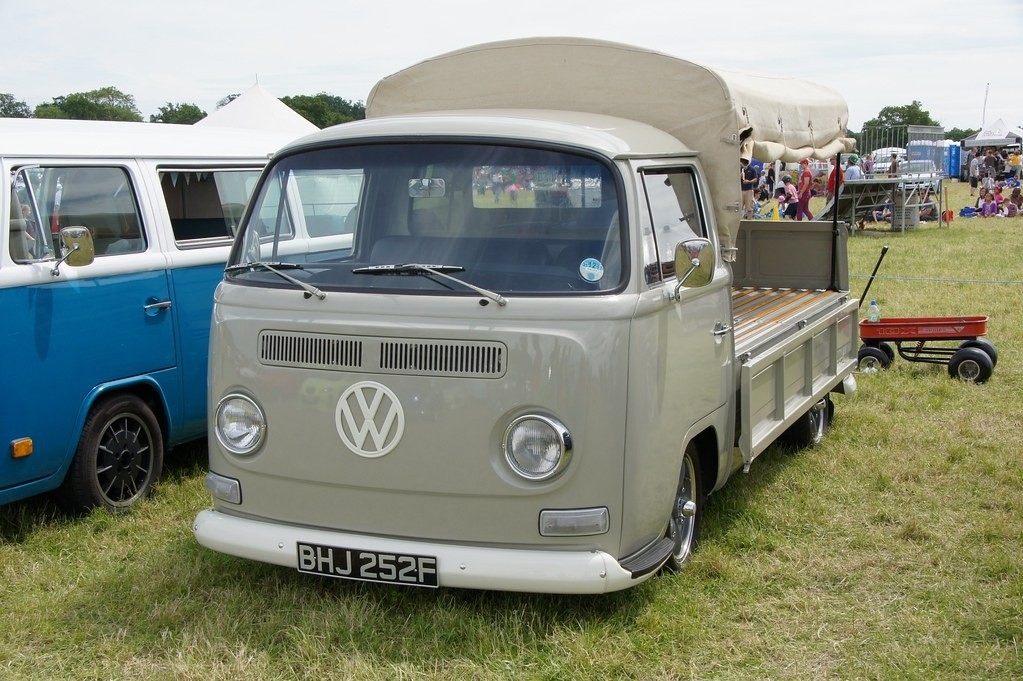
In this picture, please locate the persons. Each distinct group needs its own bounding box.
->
[778,159,814,221]
[868,189,901,222]
[864,156,874,179]
[919,189,938,221]
[741,162,775,219]
[845,154,866,180]
[826,154,843,204]
[18,175,65,235]
[965,148,1023,196]
[975,185,1023,218]
[475,166,534,205]
[888,153,900,178]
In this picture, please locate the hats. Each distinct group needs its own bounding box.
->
[850,155,858,164]
[778,195,785,203]
[975,152,982,157]
[799,159,809,164]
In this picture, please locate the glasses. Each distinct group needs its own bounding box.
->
[830,159,835,161]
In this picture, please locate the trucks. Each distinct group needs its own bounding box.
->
[182,35,860,594]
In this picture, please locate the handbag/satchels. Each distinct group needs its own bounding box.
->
[942,210,953,221]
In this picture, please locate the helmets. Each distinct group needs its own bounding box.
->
[782,174,792,183]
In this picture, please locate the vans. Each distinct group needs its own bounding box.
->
[0,115,365,522]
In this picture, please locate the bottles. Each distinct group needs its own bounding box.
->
[867,300,881,323]
[773,205,780,220]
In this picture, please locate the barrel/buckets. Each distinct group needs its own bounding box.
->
[891,190,921,231]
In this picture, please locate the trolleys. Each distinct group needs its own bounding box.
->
[851,245,999,385]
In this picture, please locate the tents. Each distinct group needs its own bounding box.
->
[961,118,1022,151]
[195,84,363,216]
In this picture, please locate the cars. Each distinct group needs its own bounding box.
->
[896,160,941,194]
[871,154,906,174]
